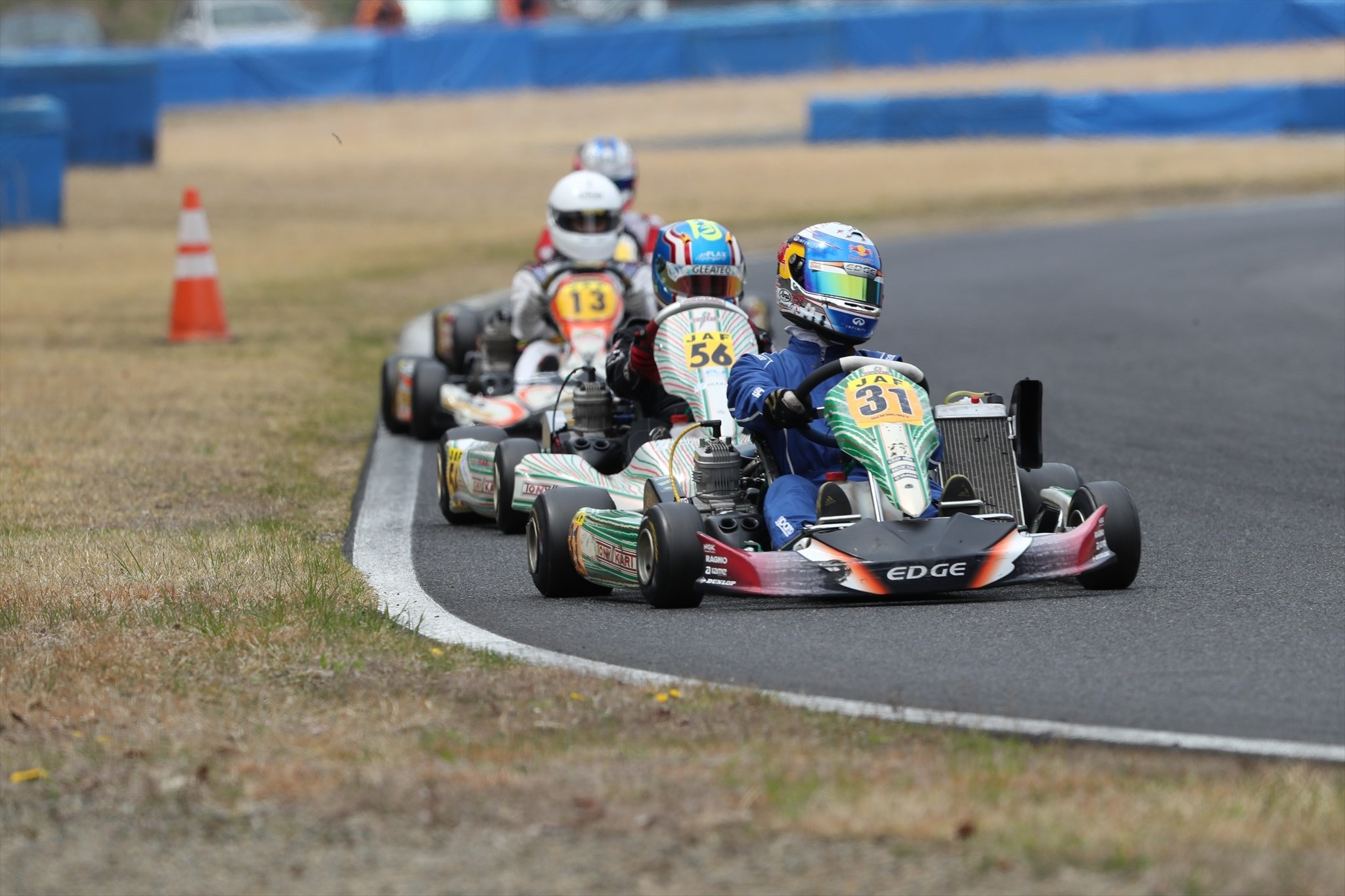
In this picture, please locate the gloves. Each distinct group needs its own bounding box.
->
[523,288,551,322]
[628,329,654,378]
[763,388,814,430]
[623,279,646,313]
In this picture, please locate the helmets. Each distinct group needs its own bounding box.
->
[651,218,747,316]
[776,222,884,346]
[546,169,621,260]
[572,135,637,210]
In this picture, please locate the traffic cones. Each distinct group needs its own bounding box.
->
[154,186,245,346]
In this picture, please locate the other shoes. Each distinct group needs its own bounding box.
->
[816,482,852,530]
[939,474,980,518]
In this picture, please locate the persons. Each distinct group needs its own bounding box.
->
[606,218,777,459]
[497,0,548,25]
[355,0,405,35]
[508,133,669,376]
[726,222,981,551]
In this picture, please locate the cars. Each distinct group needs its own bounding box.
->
[154,0,326,43]
[349,0,667,31]
[0,11,125,45]
